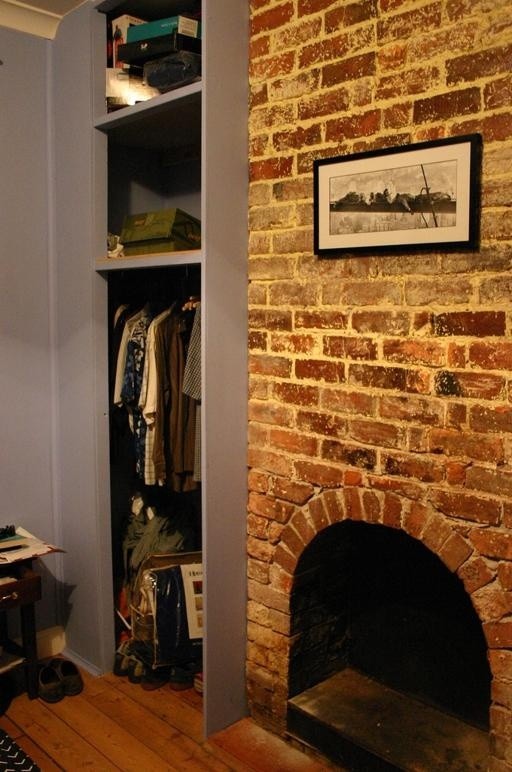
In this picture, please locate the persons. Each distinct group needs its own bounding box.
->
[382,189,415,215]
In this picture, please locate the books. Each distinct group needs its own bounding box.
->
[0,532,30,551]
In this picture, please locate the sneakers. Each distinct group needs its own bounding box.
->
[40,656,83,696]
[20,662,65,703]
[113,638,203,691]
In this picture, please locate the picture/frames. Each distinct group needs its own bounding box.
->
[312,132,484,258]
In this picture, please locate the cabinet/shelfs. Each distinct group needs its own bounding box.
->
[92,1,250,743]
[0,556,43,701]
